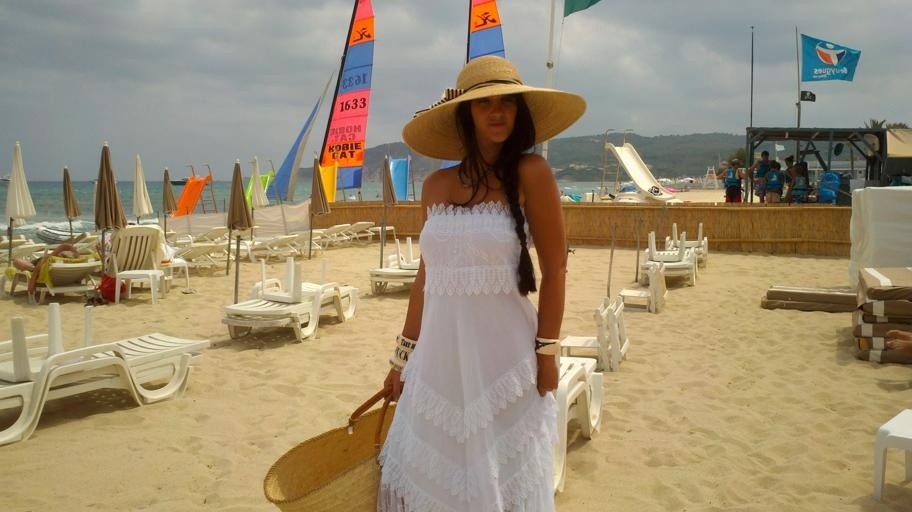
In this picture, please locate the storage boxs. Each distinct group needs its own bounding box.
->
[762,266,911,362]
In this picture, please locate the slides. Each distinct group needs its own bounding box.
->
[318,161,338,204]
[604,142,675,203]
[168,174,211,219]
[245,169,274,209]
[387,154,409,201]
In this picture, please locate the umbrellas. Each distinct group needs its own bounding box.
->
[306,153,334,260]
[132,152,153,225]
[378,153,398,269]
[3,139,37,268]
[224,157,256,304]
[162,166,179,242]
[92,139,128,275]
[61,164,82,247]
[248,155,269,242]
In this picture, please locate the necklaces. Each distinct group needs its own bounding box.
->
[455,159,494,190]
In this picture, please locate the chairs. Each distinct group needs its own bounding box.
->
[560,295,630,373]
[664,237,710,269]
[617,261,669,314]
[0,332,212,445]
[221,284,357,340]
[1,219,424,307]
[554,355,605,494]
[639,249,699,287]
[873,407,911,502]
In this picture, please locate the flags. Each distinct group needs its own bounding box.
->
[800,32,862,83]
[800,90,817,102]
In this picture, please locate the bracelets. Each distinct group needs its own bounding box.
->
[534,336,562,356]
[387,332,418,377]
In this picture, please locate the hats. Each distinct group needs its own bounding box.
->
[403,54,586,162]
[731,159,744,166]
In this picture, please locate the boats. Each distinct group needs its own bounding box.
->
[2,174,29,187]
[171,176,209,188]
[35,221,119,246]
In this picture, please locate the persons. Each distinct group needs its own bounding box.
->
[374,52,592,512]
[747,150,773,203]
[759,161,791,203]
[783,154,796,203]
[788,163,810,202]
[716,158,745,202]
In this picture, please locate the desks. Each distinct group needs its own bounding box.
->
[1,303,96,382]
[672,222,703,247]
[646,231,687,262]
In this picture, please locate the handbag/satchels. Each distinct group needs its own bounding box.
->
[263,382,397,512]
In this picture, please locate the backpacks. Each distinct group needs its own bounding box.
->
[766,170,782,190]
[724,167,741,189]
